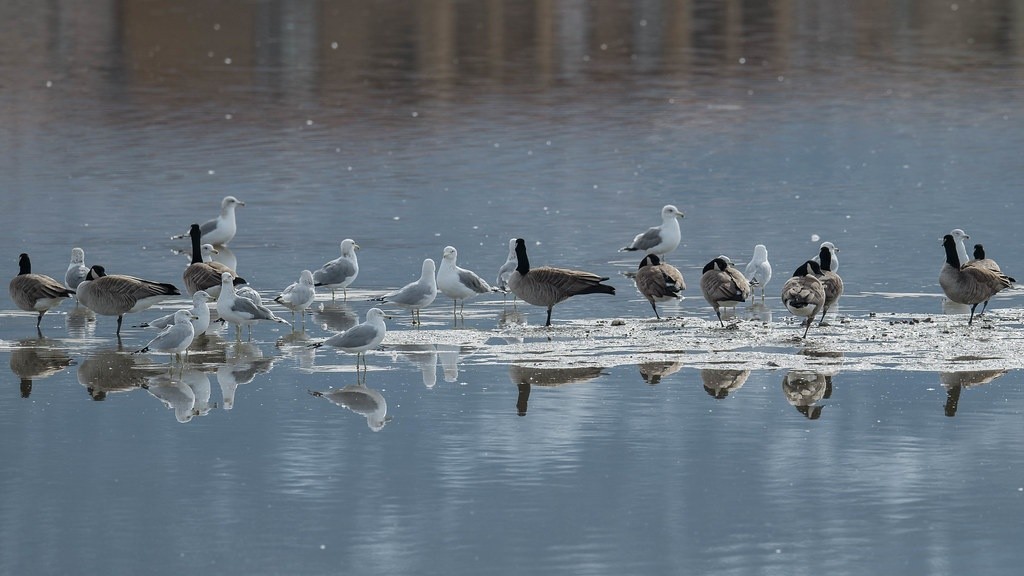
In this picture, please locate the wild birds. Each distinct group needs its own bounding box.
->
[943,355,1004,418]
[507,238,615,325]
[389,330,491,387]
[10,252,76,326]
[309,307,391,368]
[65,246,90,302]
[633,255,685,319]
[436,246,506,315]
[130,220,294,342]
[76,265,182,335]
[781,242,843,338]
[619,204,684,261]
[940,228,1016,324]
[496,239,519,310]
[745,244,772,298]
[503,361,610,417]
[308,368,392,433]
[312,238,361,297]
[131,309,199,368]
[638,349,830,422]
[7,326,321,424]
[701,255,750,327]
[275,269,317,318]
[171,196,247,246]
[379,259,437,325]
